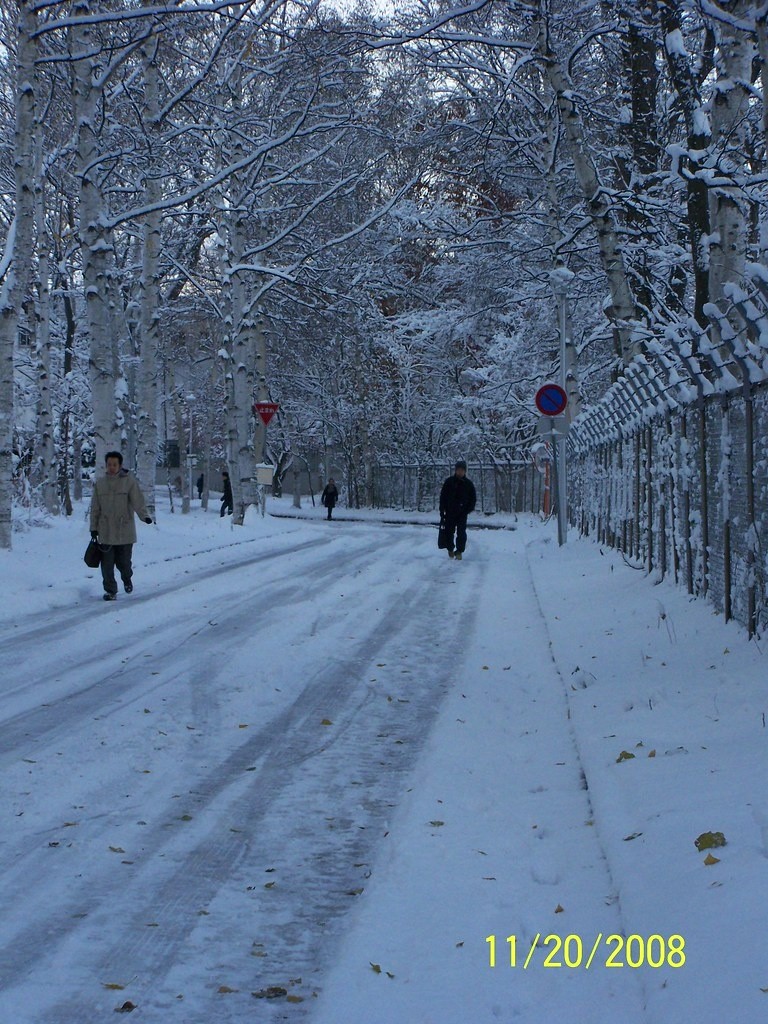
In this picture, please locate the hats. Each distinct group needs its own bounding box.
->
[455,460,467,472]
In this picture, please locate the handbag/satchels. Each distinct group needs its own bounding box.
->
[83,536,100,568]
[438,518,448,549]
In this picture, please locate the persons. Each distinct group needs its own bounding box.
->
[219,472,233,517]
[439,461,476,560]
[89,451,152,601]
[321,478,338,520]
[197,473,204,499]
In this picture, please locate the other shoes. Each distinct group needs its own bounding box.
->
[448,549,454,558]
[124,579,133,594]
[103,593,117,601]
[454,550,462,560]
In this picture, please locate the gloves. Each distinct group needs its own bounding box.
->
[91,531,98,538]
[145,518,152,524]
[220,497,223,501]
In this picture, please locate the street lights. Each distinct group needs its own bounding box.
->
[186,393,196,500]
[551,266,575,543]
